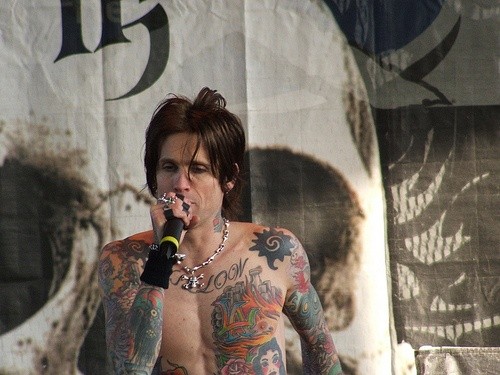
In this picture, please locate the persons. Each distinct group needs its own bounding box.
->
[99,86,346,375]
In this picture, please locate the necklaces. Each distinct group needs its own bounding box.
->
[171,217,230,289]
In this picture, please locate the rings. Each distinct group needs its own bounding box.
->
[158,192,177,205]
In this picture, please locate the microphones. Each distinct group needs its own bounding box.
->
[160,219,185,262]
